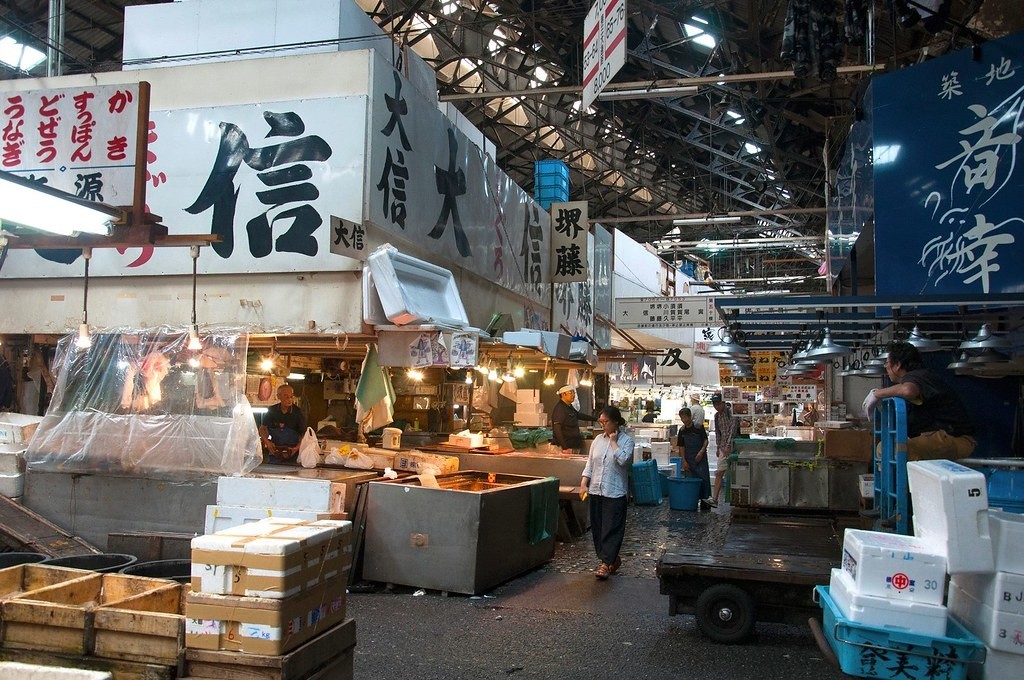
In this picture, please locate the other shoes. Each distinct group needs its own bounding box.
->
[880,514,897,527]
[859,505,881,519]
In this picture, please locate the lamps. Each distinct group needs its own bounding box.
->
[674,216,742,224]
[187,247,201,350]
[722,1,985,198]
[76,249,91,348]
[707,324,1013,377]
[0,170,128,238]
[465,349,593,386]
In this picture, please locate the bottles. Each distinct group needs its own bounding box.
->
[454,412,458,420]
[413,418,419,432]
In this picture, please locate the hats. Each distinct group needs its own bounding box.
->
[690,393,700,401]
[710,393,722,401]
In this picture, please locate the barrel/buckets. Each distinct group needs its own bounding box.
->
[469,413,492,432]
[379,428,402,449]
[666,477,703,510]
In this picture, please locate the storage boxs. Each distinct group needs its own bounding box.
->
[535,160,569,212]
[0,378,1024,680]
[680,262,694,277]
[363,247,600,365]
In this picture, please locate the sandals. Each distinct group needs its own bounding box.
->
[595,563,610,578]
[607,557,621,572]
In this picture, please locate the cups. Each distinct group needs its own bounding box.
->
[343,378,357,394]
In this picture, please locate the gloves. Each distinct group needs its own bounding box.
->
[865,395,879,417]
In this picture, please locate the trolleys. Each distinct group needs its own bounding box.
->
[654,395,909,644]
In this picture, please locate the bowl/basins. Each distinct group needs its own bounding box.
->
[986,471,1024,514]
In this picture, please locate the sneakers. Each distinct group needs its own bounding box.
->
[702,496,718,508]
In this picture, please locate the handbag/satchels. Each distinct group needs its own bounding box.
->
[296,426,373,470]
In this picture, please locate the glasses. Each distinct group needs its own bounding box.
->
[883,359,894,368]
[597,417,613,424]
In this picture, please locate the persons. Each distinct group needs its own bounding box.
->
[258,384,306,462]
[578,406,635,578]
[551,385,598,455]
[676,408,711,499]
[689,393,705,425]
[701,393,741,508]
[859,341,983,529]
